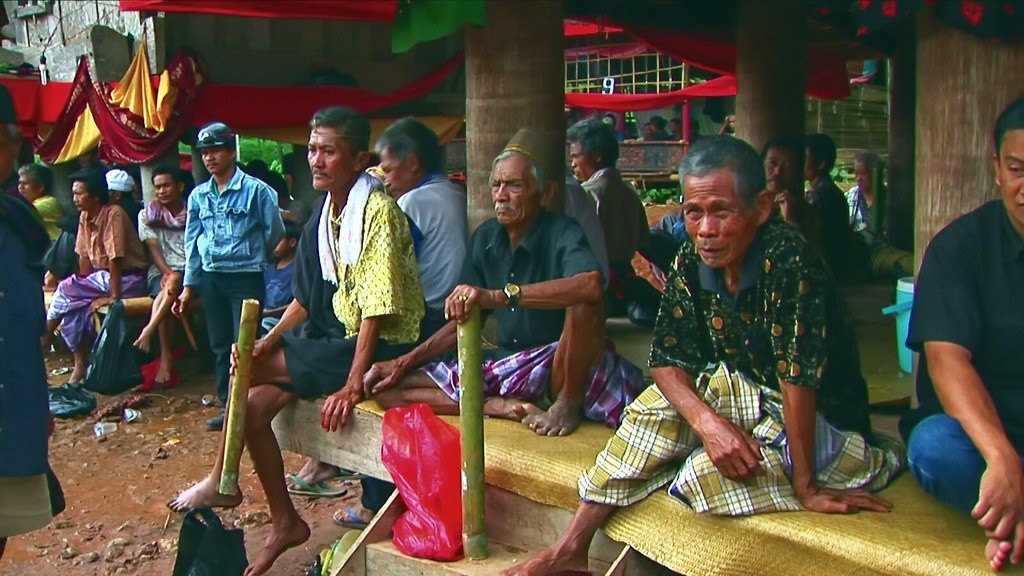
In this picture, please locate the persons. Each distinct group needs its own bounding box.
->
[164,104,426,576]
[492,133,908,576]
[718,113,735,136]
[362,127,646,436]
[285,117,467,531]
[43,167,148,383]
[567,118,687,326]
[603,112,699,144]
[844,152,884,239]
[800,132,866,283]
[43,143,144,292]
[16,163,65,246]
[170,122,285,434]
[0,82,65,560]
[898,97,1024,572]
[132,162,194,384]
[760,135,825,258]
[232,153,318,341]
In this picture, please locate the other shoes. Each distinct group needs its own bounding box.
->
[206,413,223,430]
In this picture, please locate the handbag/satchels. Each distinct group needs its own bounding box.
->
[379,400,463,560]
[172,505,249,576]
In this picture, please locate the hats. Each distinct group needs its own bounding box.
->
[0,84,19,124]
[602,113,617,126]
[501,126,562,170]
[106,169,134,192]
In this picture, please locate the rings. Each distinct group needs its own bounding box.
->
[457,295,468,302]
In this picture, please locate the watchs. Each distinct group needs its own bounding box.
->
[502,281,522,312]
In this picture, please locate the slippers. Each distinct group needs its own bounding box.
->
[333,468,366,482]
[284,473,347,498]
[334,507,368,528]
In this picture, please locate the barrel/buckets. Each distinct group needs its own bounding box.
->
[881,277,918,375]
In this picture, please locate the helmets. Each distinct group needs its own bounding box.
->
[196,123,236,150]
